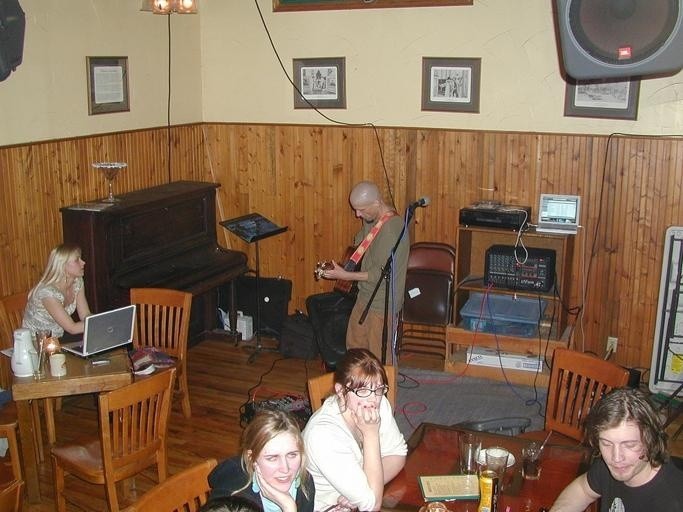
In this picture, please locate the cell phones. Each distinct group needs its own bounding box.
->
[91,359,111,364]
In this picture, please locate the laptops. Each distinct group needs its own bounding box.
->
[535,193,581,235]
[61,302,137,360]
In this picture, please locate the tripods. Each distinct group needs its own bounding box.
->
[241,243,281,365]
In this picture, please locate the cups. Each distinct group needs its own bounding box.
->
[486,443,507,488]
[456,432,480,477]
[33,329,67,381]
[519,438,544,481]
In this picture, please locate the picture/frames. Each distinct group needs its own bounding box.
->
[293,56,346,108]
[422,56,482,113]
[85,55,130,115]
[563,77,640,122]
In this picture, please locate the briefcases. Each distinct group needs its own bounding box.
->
[222,268,289,336]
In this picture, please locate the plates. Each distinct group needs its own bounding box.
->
[474,447,516,469]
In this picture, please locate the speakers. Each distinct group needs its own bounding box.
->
[553,1,683,82]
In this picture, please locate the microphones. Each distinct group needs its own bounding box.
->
[412,197,430,208]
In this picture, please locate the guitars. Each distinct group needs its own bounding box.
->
[312,245,359,295]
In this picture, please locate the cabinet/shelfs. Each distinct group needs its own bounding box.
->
[446,226,576,390]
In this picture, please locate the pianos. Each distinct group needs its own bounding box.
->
[59,179,252,348]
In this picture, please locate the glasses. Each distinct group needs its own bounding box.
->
[345,387,387,397]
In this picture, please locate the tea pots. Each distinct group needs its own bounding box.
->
[10,328,39,377]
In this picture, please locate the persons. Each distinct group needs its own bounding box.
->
[439,73,463,98]
[321,179,410,375]
[20,242,97,342]
[208,409,315,512]
[546,387,683,512]
[300,347,408,512]
[194,493,264,512]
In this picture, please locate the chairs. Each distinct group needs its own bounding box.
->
[520,345,630,450]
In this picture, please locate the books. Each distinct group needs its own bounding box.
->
[415,473,480,503]
[126,345,180,376]
